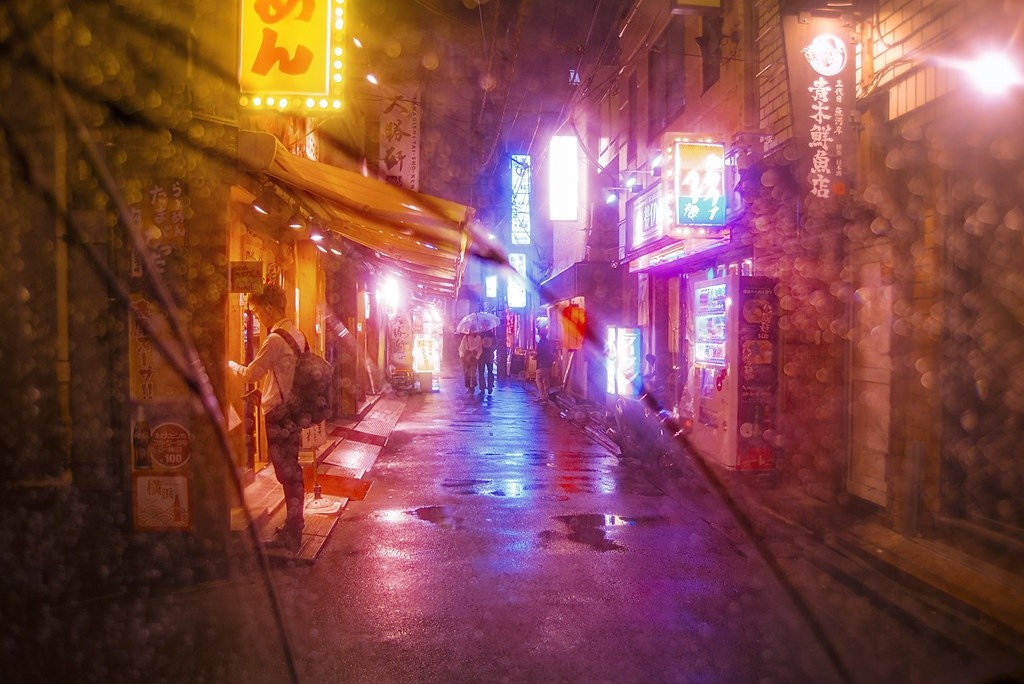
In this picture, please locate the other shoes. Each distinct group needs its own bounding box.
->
[260,531,301,552]
[478,391,485,396]
[276,523,303,536]
[488,388,492,394]
[534,396,548,404]
[465,388,475,394]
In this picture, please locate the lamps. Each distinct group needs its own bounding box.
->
[252,179,276,215]
[601,185,642,203]
[620,167,661,186]
[695,31,737,49]
[287,203,343,254]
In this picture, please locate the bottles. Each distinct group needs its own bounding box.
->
[173,494,181,521]
[133,407,152,470]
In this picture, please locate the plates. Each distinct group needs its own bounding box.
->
[743,299,772,322]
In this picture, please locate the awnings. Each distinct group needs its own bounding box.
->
[232,130,474,299]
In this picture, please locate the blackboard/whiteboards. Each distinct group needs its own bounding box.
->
[507,355,526,376]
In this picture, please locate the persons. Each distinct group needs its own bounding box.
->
[457,326,484,394]
[639,354,672,388]
[478,319,498,393]
[226,283,317,548]
[528,327,555,403]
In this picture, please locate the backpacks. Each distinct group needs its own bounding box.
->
[272,328,334,429]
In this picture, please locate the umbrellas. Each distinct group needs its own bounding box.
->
[453,310,501,335]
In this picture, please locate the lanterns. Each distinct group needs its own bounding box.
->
[561,302,586,353]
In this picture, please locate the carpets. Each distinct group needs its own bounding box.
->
[330,427,388,447]
[302,466,374,500]
